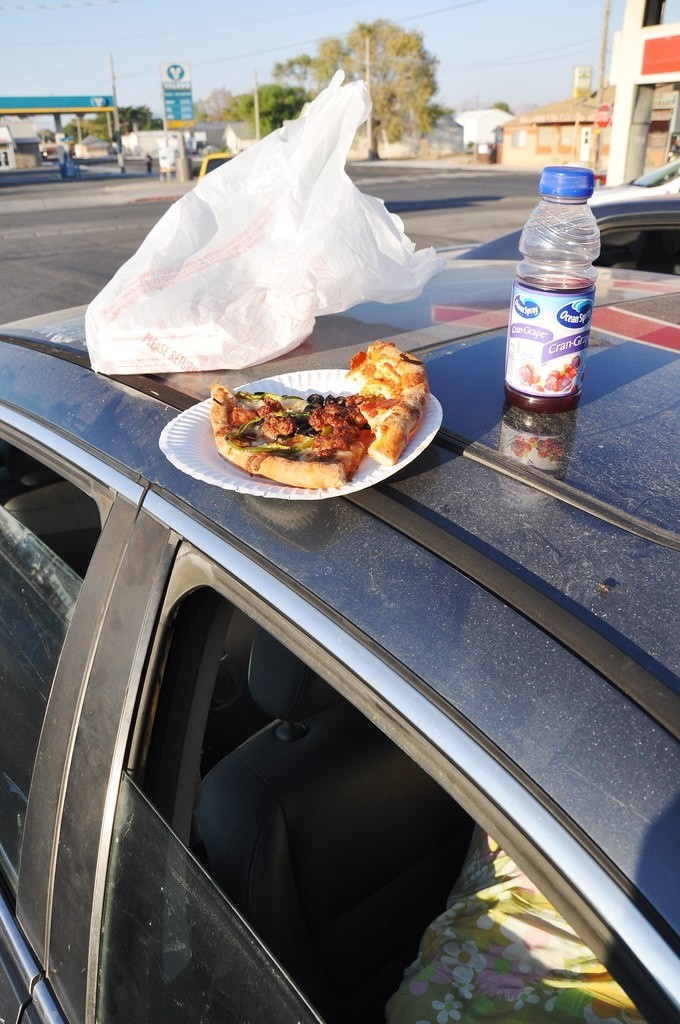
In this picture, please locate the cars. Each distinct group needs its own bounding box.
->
[0,267,679,1024]
[576,154,680,204]
[426,197,679,280]
[195,152,239,189]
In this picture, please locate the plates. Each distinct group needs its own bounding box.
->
[157,369,444,502]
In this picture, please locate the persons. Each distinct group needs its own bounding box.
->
[145,154,153,173]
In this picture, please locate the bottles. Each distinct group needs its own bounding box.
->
[502,165,601,408]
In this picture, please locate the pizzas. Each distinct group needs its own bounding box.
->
[207,382,369,489]
[344,339,430,468]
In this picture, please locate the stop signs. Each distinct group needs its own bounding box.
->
[595,105,613,128]
